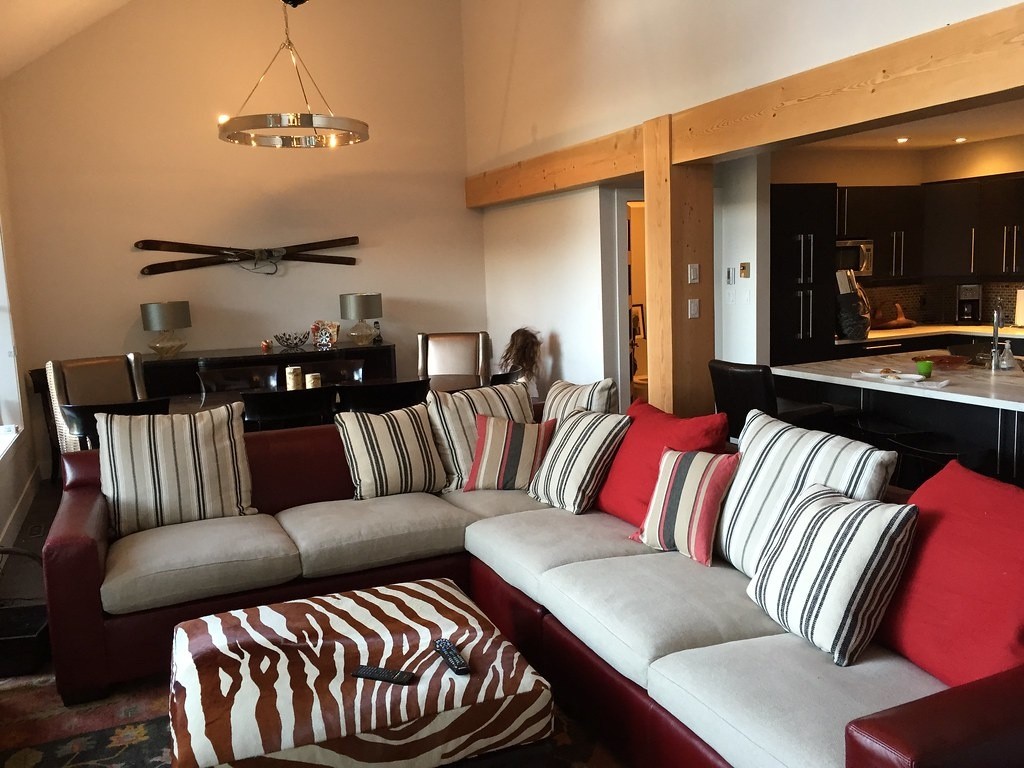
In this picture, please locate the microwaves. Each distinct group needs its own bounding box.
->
[835,240,873,276]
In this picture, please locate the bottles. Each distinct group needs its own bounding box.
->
[997,309,1005,328]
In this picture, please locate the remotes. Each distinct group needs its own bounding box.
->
[432,638,469,675]
[350,665,415,685]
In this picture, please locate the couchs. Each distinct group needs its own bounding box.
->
[464,443,1024,768]
[42,395,547,706]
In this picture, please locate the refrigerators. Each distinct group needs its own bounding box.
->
[769,182,839,368]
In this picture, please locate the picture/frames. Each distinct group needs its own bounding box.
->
[630,303,645,340]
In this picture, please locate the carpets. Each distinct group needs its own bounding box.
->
[0,663,596,768]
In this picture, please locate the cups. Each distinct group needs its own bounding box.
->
[286,367,301,391]
[306,373,321,388]
[917,361,932,377]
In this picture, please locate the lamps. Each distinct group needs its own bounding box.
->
[139,299,192,359]
[340,292,383,344]
[218,0,369,148]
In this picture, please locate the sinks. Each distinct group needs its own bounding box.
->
[966,352,1024,367]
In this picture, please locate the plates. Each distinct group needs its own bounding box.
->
[860,369,902,377]
[881,374,925,382]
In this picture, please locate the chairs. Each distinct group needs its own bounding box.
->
[709,358,832,446]
[45,330,523,454]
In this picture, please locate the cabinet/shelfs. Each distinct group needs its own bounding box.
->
[770,170,1024,367]
[28,339,397,486]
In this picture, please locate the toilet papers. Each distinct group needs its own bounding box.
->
[1015,290,1024,326]
[835,270,850,294]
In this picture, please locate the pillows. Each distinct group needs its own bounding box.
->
[94,401,258,541]
[332,377,1024,689]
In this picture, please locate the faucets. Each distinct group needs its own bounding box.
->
[990,305,1004,370]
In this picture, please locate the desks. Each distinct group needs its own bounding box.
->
[769,348,1024,478]
[168,576,555,768]
[168,374,496,415]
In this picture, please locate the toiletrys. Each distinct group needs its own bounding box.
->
[999,340,1016,369]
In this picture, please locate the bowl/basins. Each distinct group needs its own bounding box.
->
[274,330,310,348]
